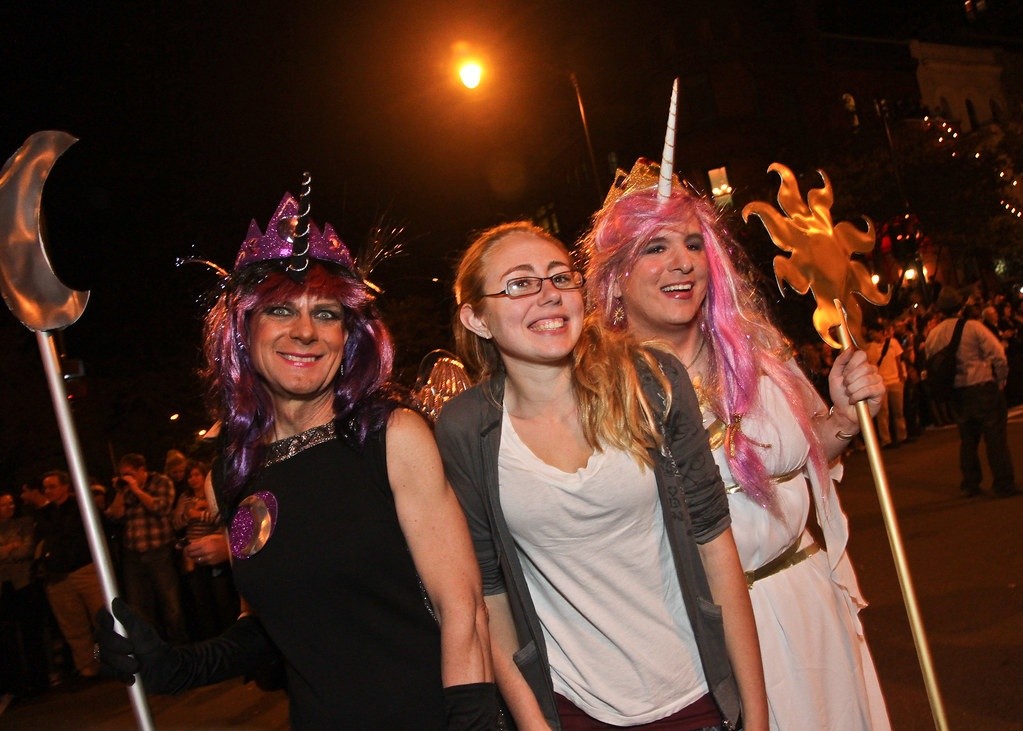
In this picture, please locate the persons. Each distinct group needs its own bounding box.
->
[94,192,501,731]
[728,116,1023,458]
[925,285,1023,497]
[583,154,890,731]
[0,347,478,715]
[434,219,772,731]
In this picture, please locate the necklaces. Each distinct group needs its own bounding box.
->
[687,339,704,369]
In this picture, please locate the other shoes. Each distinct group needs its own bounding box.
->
[936,411,960,426]
[995,484,1023,498]
[961,482,992,496]
[52,671,102,691]
[883,442,894,450]
[900,437,910,443]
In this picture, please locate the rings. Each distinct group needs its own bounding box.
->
[88,641,101,663]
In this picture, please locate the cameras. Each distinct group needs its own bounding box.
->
[116,479,128,488]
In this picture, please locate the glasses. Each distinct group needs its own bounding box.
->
[481,269,585,300]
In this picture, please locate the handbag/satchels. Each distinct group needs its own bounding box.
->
[924,347,956,398]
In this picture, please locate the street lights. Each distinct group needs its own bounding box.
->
[459,57,606,206]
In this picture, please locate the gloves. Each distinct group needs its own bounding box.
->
[98,598,194,696]
[441,681,509,731]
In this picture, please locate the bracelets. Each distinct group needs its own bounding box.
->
[826,406,856,441]
